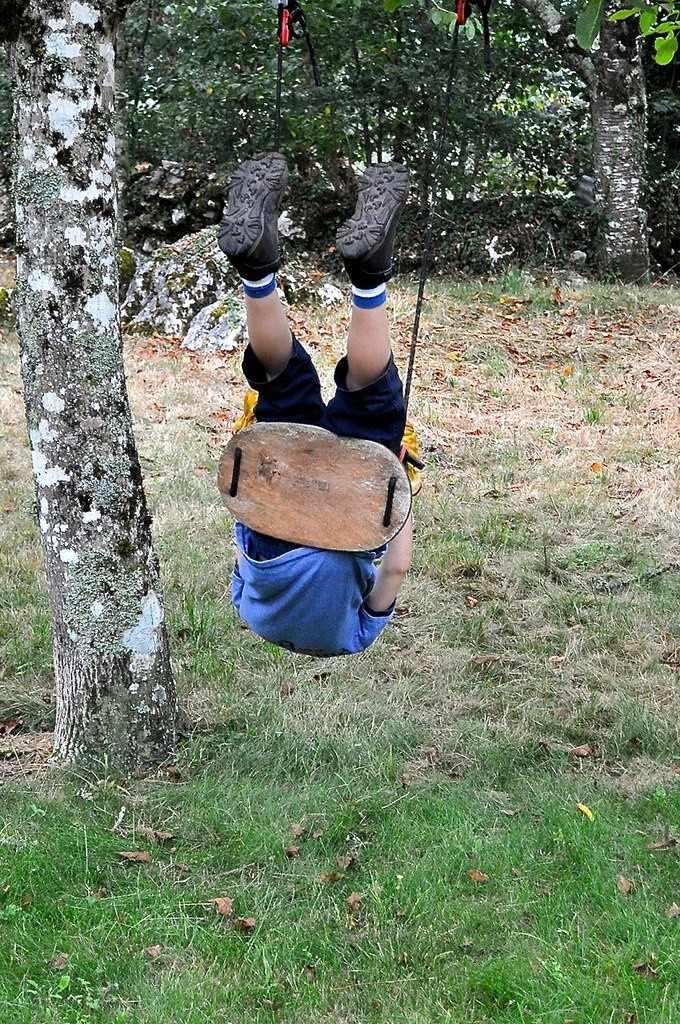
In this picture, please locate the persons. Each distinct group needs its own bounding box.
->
[216,149,417,659]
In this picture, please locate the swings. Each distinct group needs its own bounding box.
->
[219,1,467,556]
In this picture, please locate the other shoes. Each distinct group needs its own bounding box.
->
[220,152,292,281]
[336,161,410,291]
